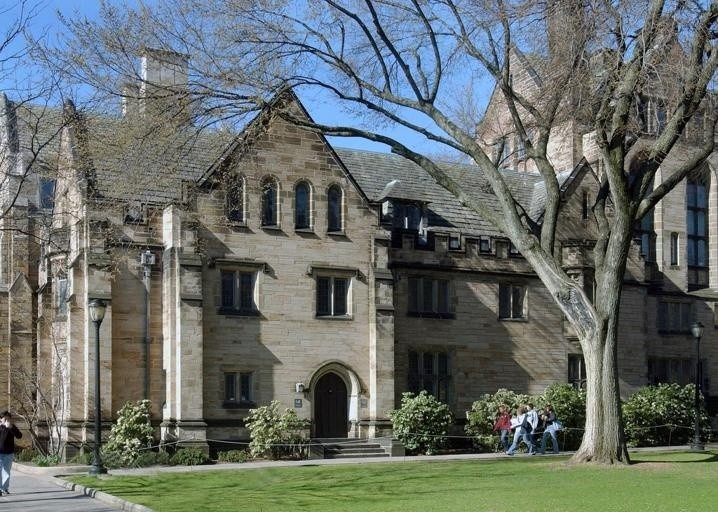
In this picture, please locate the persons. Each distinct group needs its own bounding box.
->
[0,411,23,496]
[521,403,539,452]
[493,405,510,455]
[509,406,534,455]
[537,405,558,453]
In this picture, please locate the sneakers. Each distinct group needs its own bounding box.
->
[552,450,559,454]
[529,448,545,455]
[507,452,514,455]
[0,488,9,496]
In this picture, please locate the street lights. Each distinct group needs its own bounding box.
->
[86,297,110,478]
[686,319,706,451]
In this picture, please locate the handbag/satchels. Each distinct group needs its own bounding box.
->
[552,420,564,430]
[526,422,532,433]
